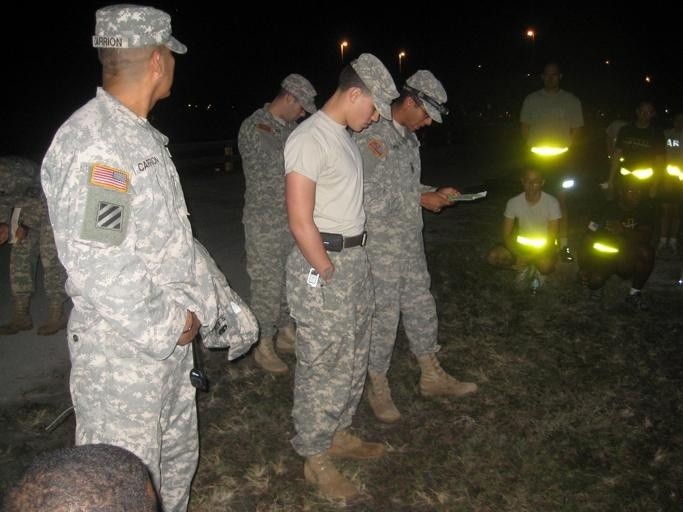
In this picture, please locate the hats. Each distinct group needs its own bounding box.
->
[280,73,317,116]
[351,52,400,121]
[87,3,188,54]
[402,69,450,125]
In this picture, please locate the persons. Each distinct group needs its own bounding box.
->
[573,97,680,311]
[519,59,585,199]
[347,67,481,425]
[0,441,163,512]
[280,51,401,504]
[0,154,73,338]
[42,3,218,512]
[233,72,323,378]
[486,163,566,285]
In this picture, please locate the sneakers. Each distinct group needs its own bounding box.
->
[513,265,531,292]
[584,286,604,317]
[654,235,682,261]
[533,267,549,291]
[624,291,652,315]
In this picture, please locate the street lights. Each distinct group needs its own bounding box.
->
[397,52,404,76]
[339,41,348,67]
[527,31,535,68]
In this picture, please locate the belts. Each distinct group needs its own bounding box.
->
[340,231,368,249]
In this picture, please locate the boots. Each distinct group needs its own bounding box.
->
[252,338,289,376]
[364,373,402,425]
[36,298,69,336]
[275,322,300,352]
[417,353,480,399]
[1,297,34,337]
[324,427,386,462]
[303,450,360,503]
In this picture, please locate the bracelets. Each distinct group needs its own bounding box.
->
[182,311,194,335]
